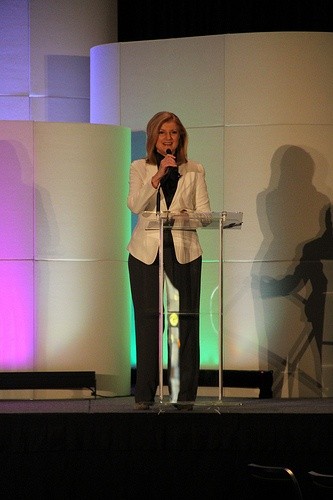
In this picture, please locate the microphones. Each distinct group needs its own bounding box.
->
[166,148,180,178]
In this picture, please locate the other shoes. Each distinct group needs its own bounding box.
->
[134,401,150,410]
[176,401,195,411]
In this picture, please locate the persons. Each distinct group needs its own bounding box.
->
[125,111,212,413]
